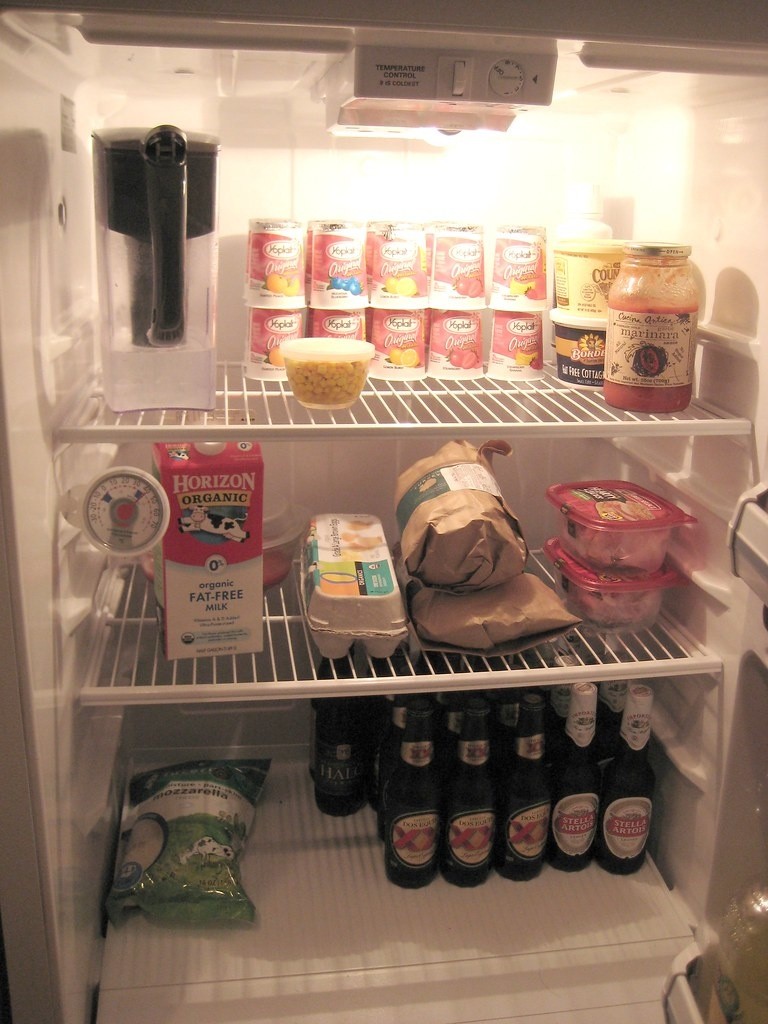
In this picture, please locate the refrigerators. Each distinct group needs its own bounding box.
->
[0,0,768,1024]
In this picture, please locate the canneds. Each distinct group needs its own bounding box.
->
[604,241,699,414]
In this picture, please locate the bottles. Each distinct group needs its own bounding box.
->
[308,628,654,888]
[603,241,700,412]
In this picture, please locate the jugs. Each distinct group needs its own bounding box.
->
[90,125,222,412]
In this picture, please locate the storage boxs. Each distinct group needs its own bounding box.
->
[540,536,680,633]
[299,513,409,659]
[547,478,699,573]
[139,471,309,591]
